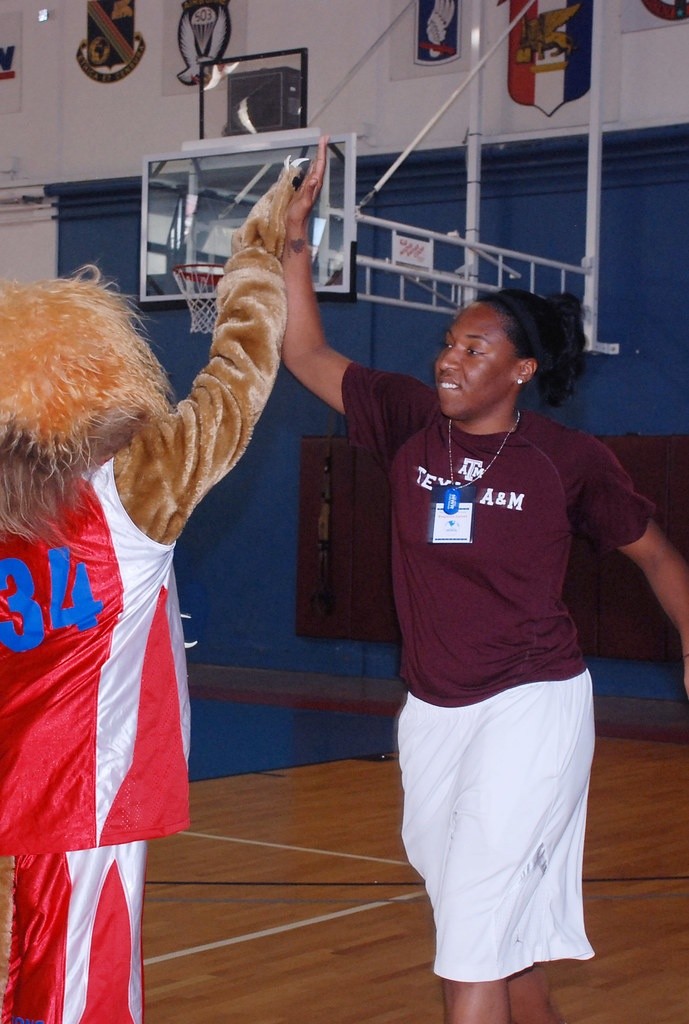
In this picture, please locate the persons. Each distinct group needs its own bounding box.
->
[280,129,689,1024]
[0,155,310,1024]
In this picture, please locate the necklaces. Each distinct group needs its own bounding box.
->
[443,409,521,515]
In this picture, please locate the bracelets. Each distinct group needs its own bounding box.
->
[282,238,311,257]
[683,653,689,660]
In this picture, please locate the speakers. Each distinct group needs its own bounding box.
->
[227,66,301,137]
